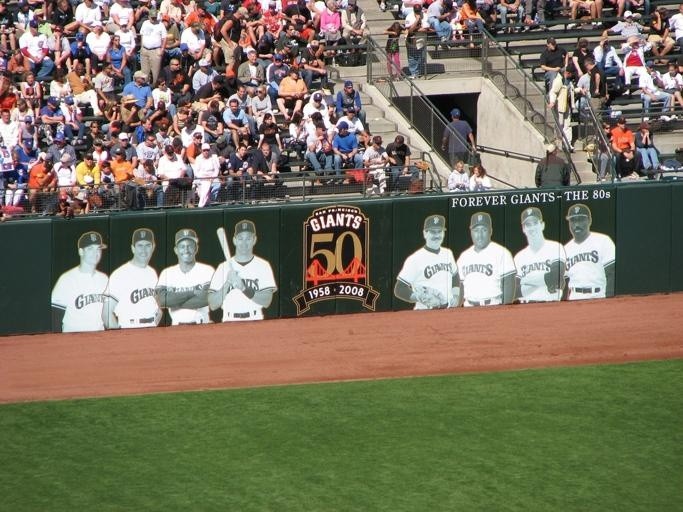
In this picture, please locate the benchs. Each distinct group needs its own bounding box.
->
[378,0,683,182]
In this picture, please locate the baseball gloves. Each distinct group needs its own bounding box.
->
[415,287,447,309]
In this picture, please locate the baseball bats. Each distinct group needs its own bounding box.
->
[216,227,238,287]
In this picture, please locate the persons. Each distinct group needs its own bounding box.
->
[511,206,567,302]
[560,202,616,301]
[459,210,518,308]
[151,228,216,329]
[205,220,277,324]
[392,214,460,312]
[99,228,161,331]
[1,0,421,221]
[400,0,683,193]
[49,231,109,334]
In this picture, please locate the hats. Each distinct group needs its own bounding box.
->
[29,20,38,28]
[77,231,106,249]
[628,36,641,44]
[75,16,129,40]
[48,96,61,108]
[131,228,155,246]
[201,143,210,150]
[64,96,74,104]
[521,207,543,225]
[133,71,147,79]
[199,59,210,67]
[336,81,356,130]
[545,143,556,153]
[174,228,199,245]
[60,152,72,163]
[451,108,460,118]
[149,3,250,27]
[17,0,26,7]
[470,212,491,228]
[234,219,255,235]
[123,94,138,104]
[624,10,632,18]
[424,215,448,232]
[313,93,322,101]
[274,39,320,73]
[179,42,189,53]
[646,60,655,67]
[24,115,64,147]
[86,132,129,159]
[565,204,591,220]
[641,120,650,129]
[394,135,404,148]
[373,136,382,145]
[618,115,626,124]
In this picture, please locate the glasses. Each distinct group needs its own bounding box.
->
[605,127,610,128]
[172,63,179,67]
[256,90,262,94]
[148,138,155,142]
[161,83,164,86]
[195,136,202,139]
